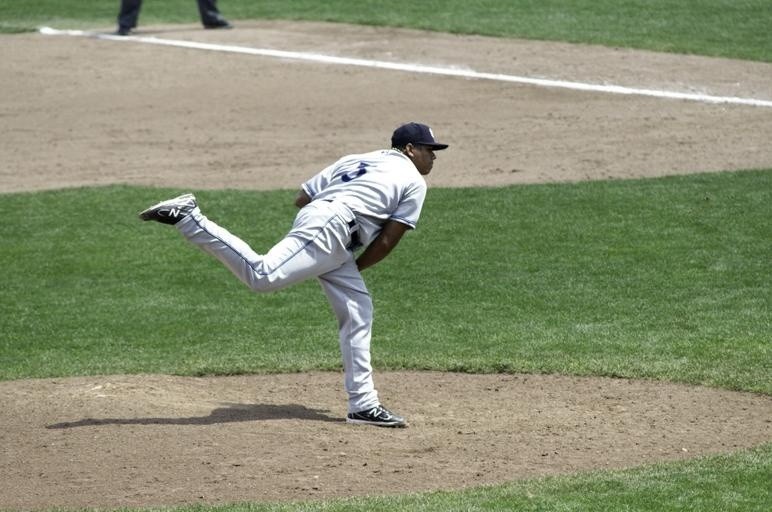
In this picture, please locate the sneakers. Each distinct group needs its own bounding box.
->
[204,17,228,29]
[139,193,196,224]
[347,405,407,427]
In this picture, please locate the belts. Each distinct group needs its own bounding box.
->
[349,220,363,251]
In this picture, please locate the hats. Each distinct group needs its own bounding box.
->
[392,122,449,151]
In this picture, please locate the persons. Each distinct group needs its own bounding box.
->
[116,0,233,36]
[136,121,450,426]
